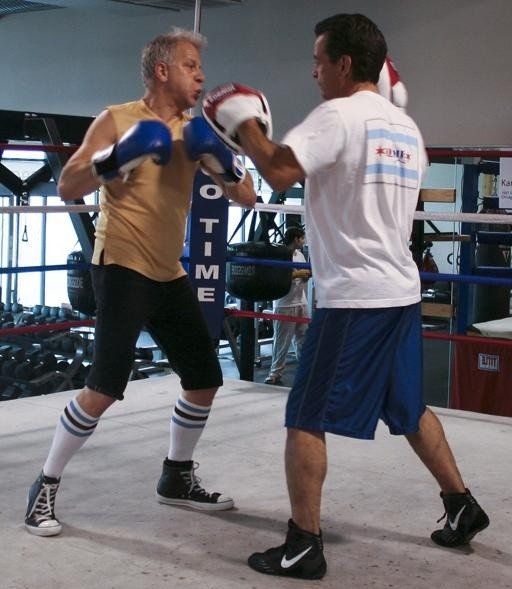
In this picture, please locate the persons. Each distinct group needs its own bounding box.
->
[22,26,260,537]
[263,227,313,384]
[201,10,492,579]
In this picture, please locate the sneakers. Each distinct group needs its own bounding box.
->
[247,518,328,581]
[264,374,281,385]
[154,456,235,512]
[24,468,63,537]
[429,489,492,549]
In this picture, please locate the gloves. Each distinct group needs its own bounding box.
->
[184,116,247,188]
[201,80,274,158]
[91,118,172,187]
[377,56,410,114]
